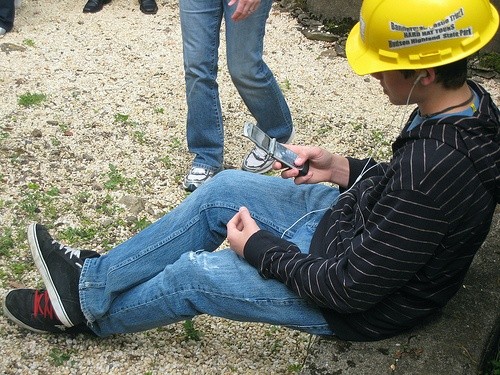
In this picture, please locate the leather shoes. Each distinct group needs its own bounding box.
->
[138,0,158,14]
[83,0,112,13]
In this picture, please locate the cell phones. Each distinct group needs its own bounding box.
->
[244,121,309,176]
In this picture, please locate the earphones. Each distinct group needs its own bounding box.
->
[413,70,427,85]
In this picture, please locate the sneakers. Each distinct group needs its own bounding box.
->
[27,222,100,328]
[240,126,295,174]
[182,165,224,192]
[3,288,99,338]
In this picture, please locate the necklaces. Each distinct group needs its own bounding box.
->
[420,86,475,119]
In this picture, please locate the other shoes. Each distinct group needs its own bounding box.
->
[0,27,6,39]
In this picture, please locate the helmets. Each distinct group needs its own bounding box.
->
[345,0,500,76]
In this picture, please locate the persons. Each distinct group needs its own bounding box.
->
[0,0,500,340]
